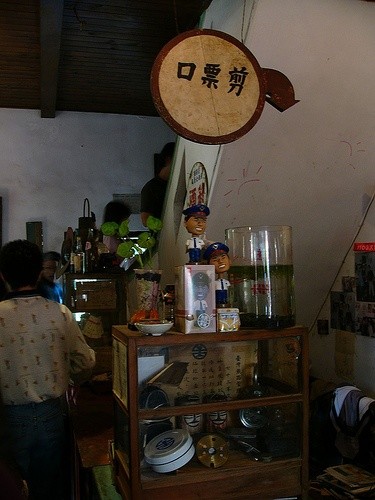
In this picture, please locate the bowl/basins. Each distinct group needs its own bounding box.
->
[135,323,174,336]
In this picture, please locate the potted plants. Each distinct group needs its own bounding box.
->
[100,216,165,311]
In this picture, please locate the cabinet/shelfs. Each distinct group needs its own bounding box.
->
[65,274,117,471]
[111,325,309,500]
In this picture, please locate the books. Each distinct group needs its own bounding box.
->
[316,464,375,500]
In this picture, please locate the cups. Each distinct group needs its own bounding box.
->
[225,226,296,329]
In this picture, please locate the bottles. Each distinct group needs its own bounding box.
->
[70,239,75,274]
[84,228,98,273]
[75,236,85,274]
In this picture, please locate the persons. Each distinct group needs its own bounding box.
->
[102,200,132,265]
[0,239,96,500]
[338,263,375,338]
[204,242,231,307]
[140,142,176,239]
[181,204,210,265]
[38,251,64,304]
